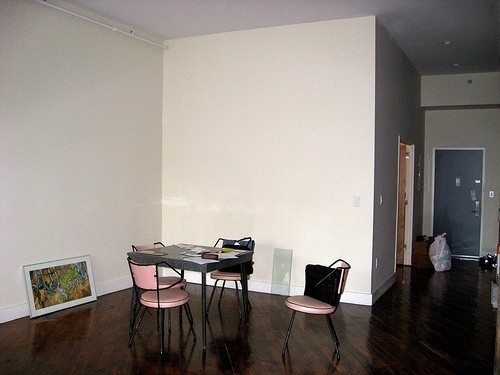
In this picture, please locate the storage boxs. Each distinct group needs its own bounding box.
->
[412,241,432,256]
[412,254,431,267]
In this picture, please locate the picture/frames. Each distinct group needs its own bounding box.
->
[23,255,97,318]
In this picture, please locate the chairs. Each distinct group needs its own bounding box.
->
[129,258,198,354]
[208,238,255,308]
[275,259,352,363]
[133,243,187,328]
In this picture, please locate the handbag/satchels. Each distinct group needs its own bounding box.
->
[429,232,452,272]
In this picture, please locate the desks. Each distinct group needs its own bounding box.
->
[123,244,254,349]
